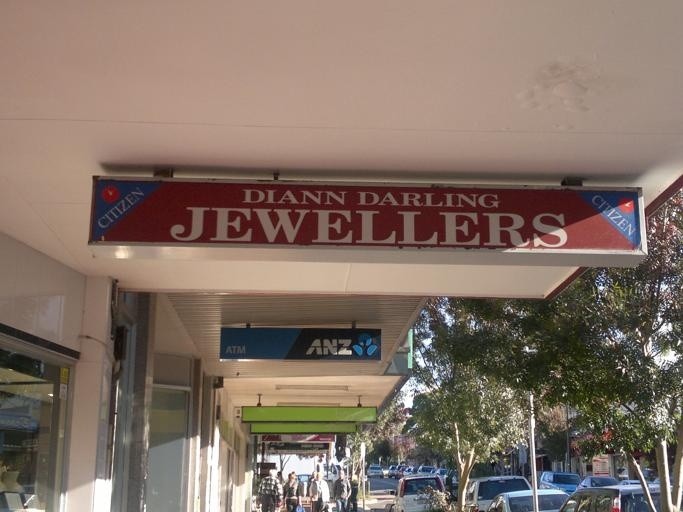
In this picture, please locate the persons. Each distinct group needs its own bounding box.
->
[256,468,358,512]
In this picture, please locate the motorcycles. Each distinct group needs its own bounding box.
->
[644,463,656,480]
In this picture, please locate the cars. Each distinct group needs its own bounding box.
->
[283,464,342,497]
[559,482,675,512]
[362,461,645,511]
